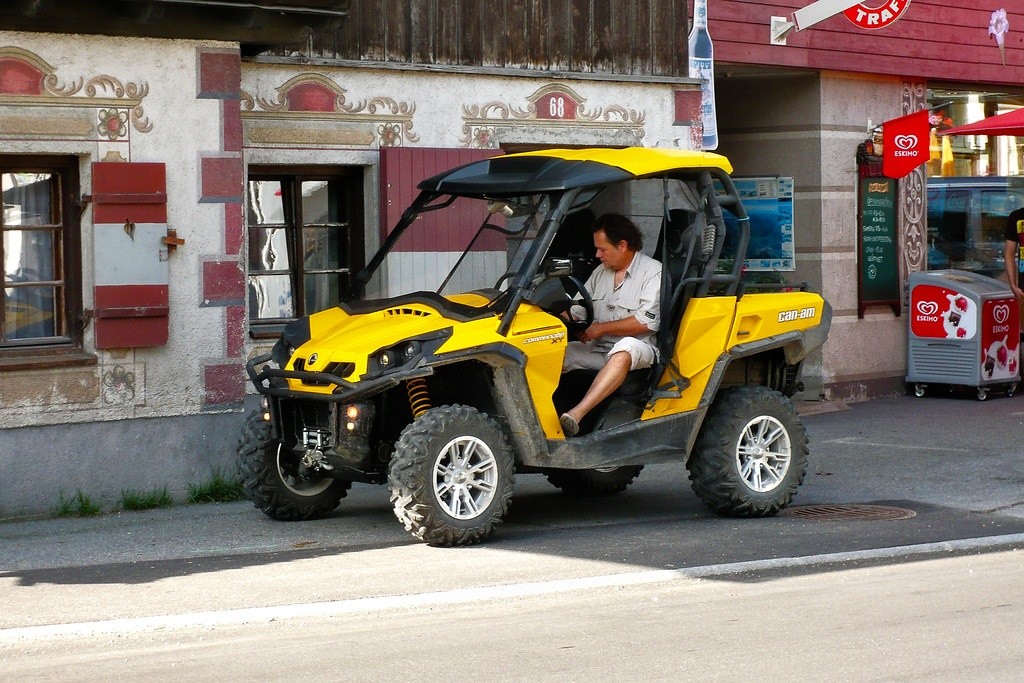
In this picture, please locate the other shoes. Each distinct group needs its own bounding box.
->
[559,412,580,437]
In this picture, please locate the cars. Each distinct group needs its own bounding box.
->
[234,148,835,546]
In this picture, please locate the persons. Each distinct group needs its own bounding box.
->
[1005,207,1024,392]
[548,211,662,437]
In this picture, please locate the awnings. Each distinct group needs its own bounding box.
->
[937,109,1024,136]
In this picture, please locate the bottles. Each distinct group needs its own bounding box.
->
[969,238,972,247]
[926,128,940,175]
[686,0,718,149]
[998,246,1003,260]
[941,136,954,176]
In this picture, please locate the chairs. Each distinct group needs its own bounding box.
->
[589,254,684,397]
